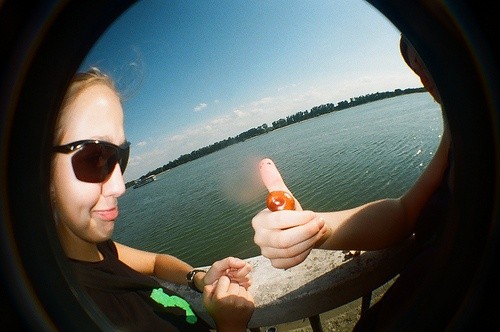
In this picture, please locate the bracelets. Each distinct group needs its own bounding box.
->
[186,269,207,294]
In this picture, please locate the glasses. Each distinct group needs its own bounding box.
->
[55,140,130,183]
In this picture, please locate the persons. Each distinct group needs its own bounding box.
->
[251,25,454,271]
[56,65,256,331]
